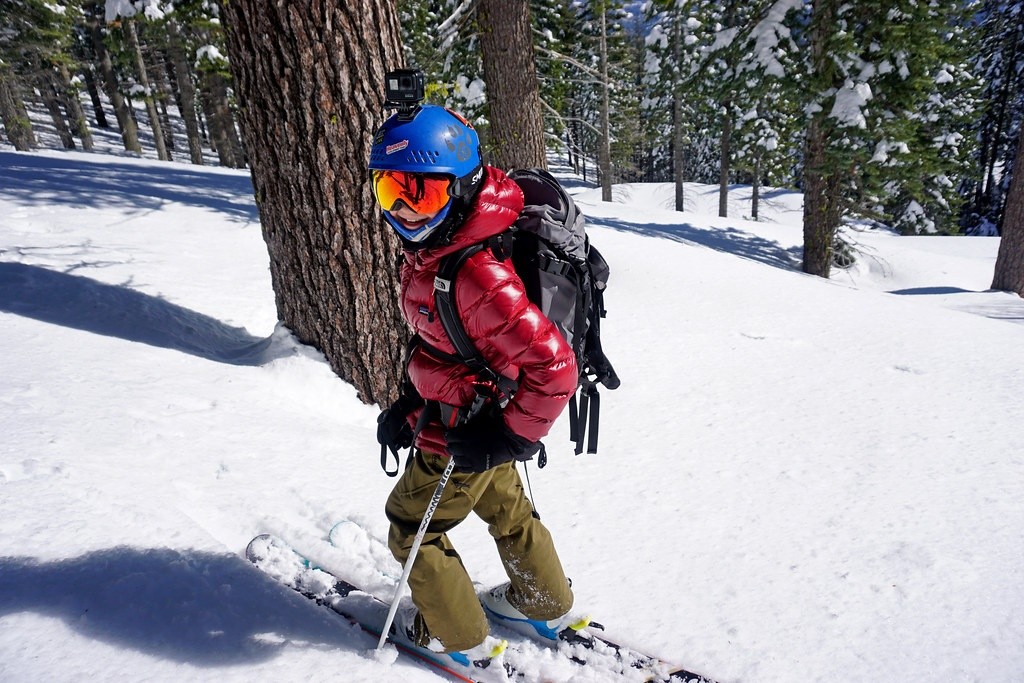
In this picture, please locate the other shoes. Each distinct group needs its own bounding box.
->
[484,582,575,630]
[399,610,494,669]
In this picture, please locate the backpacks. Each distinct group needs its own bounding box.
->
[434,167,621,456]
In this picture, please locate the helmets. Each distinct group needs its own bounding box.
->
[368,104,483,243]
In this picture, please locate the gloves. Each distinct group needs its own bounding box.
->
[444,427,511,474]
[376,409,413,450]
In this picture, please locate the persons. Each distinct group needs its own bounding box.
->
[409,174,425,203]
[369,105,605,683]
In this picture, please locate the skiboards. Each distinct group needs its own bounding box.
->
[241,517,716,683]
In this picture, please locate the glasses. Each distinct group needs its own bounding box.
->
[371,169,453,218]
